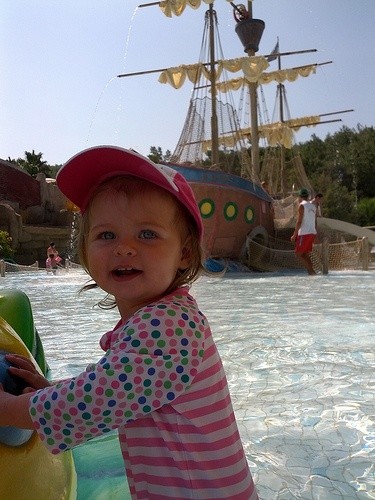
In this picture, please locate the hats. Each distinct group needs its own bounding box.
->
[55,145,203,247]
[299,188,308,196]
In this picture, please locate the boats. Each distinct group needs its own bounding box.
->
[117,0,355,273]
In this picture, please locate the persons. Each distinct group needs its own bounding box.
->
[286,186,329,276]
[44,242,65,274]
[0,144,261,500]
